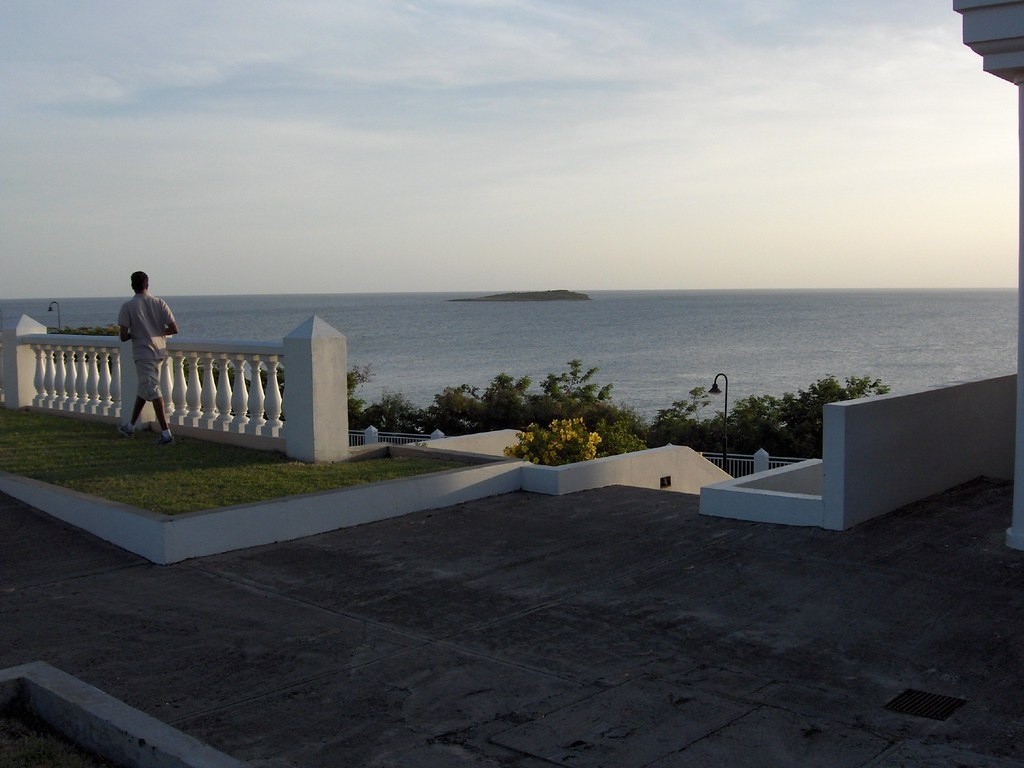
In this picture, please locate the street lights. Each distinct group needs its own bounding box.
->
[708,373,728,473]
[47,301,60,330]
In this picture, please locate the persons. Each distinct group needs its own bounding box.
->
[117,270,179,445]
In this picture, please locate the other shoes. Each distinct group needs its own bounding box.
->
[117,422,136,440]
[149,431,176,445]
